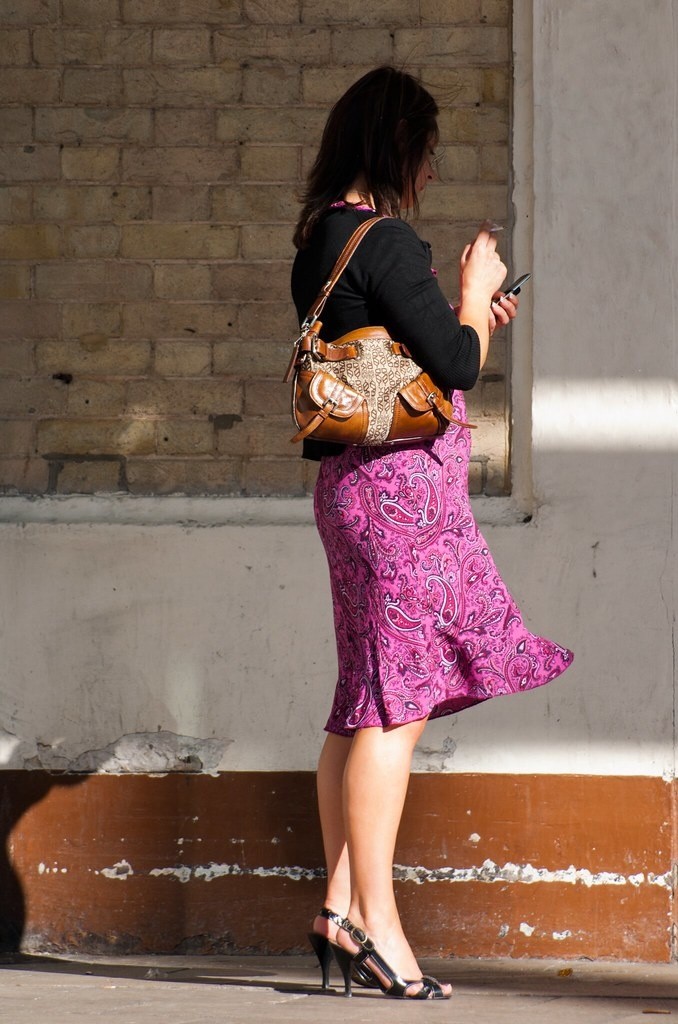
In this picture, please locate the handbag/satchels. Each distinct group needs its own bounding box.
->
[283,216,478,447]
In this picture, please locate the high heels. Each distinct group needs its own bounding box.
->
[307,907,379,989]
[328,917,454,1000]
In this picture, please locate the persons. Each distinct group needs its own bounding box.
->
[295,68,576,999]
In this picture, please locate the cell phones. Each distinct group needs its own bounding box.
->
[495,274,532,307]
